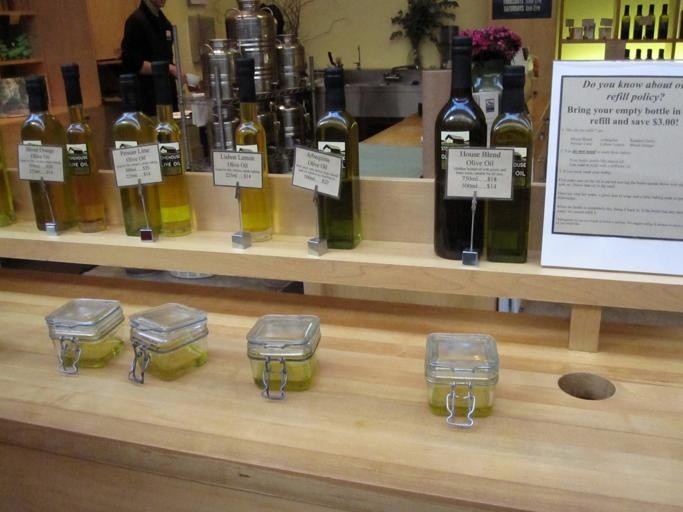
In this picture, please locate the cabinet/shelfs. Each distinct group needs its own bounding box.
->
[0,8,45,68]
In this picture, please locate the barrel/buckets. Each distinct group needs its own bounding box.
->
[199,0,310,174]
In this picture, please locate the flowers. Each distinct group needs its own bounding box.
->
[453,23,524,70]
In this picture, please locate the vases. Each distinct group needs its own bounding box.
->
[479,53,506,74]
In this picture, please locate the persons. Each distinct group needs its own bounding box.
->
[120,1,206,117]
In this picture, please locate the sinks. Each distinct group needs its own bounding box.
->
[326,67,421,120]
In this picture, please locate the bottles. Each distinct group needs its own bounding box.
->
[0,149,15,229]
[112,74,163,236]
[619,4,683,40]
[431,36,482,260]
[152,61,192,237]
[21,75,76,231]
[624,48,664,59]
[232,57,275,244]
[317,69,363,249]
[58,62,107,233]
[483,63,532,264]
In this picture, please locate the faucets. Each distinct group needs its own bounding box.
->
[392,64,419,72]
[353,44,361,69]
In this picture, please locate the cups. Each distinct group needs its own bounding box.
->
[569,28,582,39]
[599,28,610,39]
[583,22,595,39]
[440,26,457,68]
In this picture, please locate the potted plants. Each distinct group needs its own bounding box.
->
[384,0,460,86]
